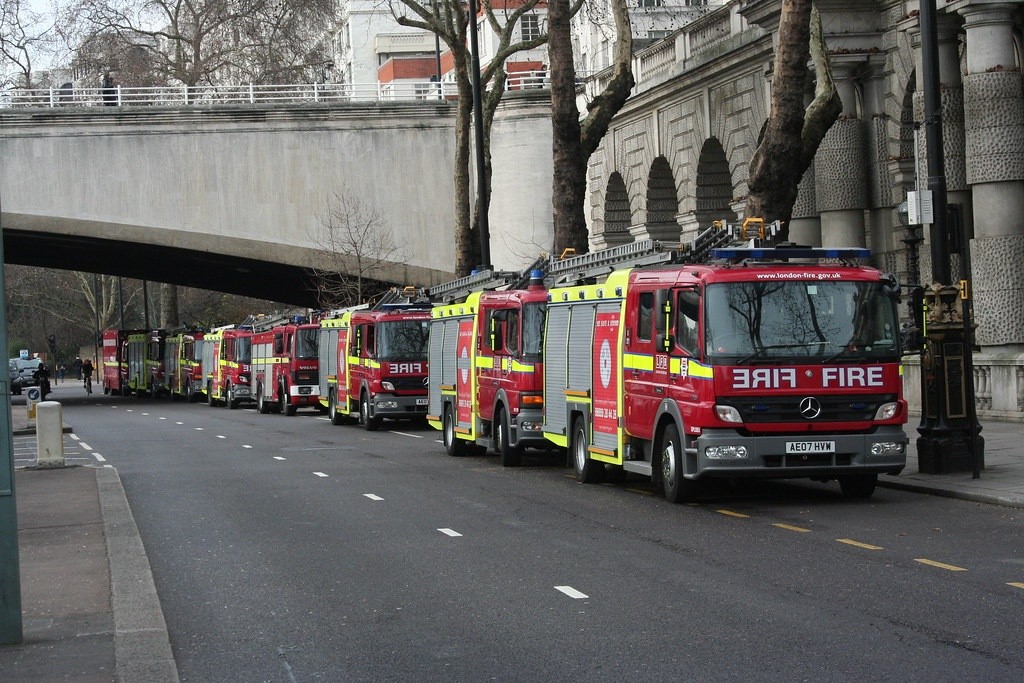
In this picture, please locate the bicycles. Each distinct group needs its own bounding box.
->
[84,376,92,396]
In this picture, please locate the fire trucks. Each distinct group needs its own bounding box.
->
[121,326,178,400]
[541,216,911,502]
[317,286,434,432]
[201,315,257,410]
[247,313,326,416]
[164,322,217,402]
[423,266,551,466]
[100,327,153,396]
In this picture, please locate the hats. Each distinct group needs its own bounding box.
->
[76,355,80,359]
[84,359,91,362]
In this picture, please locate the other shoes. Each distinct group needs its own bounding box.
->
[90,389,92,393]
[78,379,81,383]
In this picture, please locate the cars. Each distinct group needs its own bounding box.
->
[8,358,45,395]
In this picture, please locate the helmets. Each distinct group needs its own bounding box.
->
[38,363,43,369]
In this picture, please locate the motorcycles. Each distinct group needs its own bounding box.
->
[31,368,51,401]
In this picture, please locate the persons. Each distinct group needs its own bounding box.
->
[59,364,68,383]
[33,363,52,393]
[82,359,94,393]
[430,74,437,82]
[102,74,118,105]
[538,65,548,89]
[72,355,83,382]
